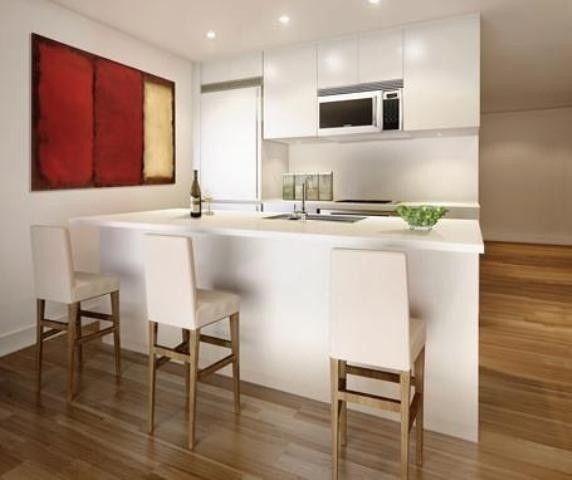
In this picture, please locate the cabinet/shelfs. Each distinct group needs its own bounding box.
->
[316,25,403,91]
[263,44,319,144]
[403,11,480,137]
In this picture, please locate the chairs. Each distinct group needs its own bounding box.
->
[29,225,121,400]
[141,234,240,450]
[329,246,426,480]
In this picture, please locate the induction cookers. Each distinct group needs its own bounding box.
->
[336,200,391,204]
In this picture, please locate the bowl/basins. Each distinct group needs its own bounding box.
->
[395,206,448,231]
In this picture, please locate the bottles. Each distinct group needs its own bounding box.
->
[282,172,333,201]
[190,169,201,217]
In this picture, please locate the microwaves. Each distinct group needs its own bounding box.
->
[317,88,402,136]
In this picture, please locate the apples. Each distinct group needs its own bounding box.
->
[394,205,447,226]
